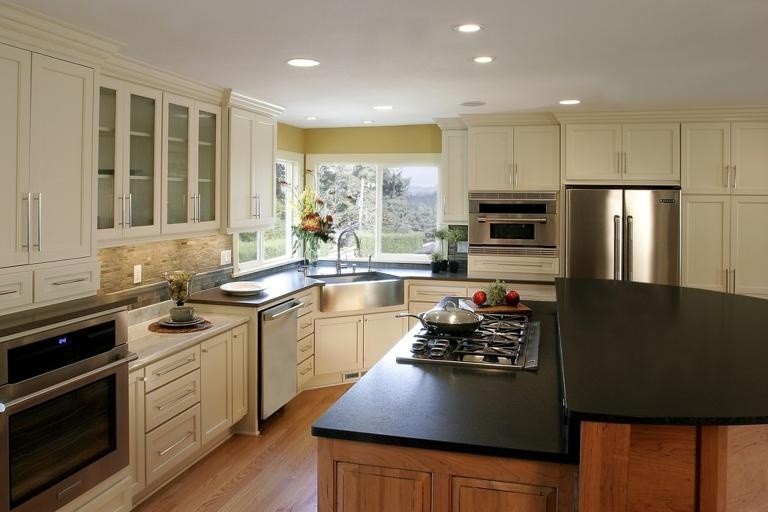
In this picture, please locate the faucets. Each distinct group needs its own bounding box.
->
[336,228,360,276]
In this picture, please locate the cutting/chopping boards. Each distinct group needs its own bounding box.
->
[459,299,532,313]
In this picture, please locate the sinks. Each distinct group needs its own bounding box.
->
[306,270,403,312]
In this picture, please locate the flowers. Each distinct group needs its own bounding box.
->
[447,229,466,262]
[165,270,193,306]
[278,168,342,269]
[435,229,449,259]
[431,252,441,262]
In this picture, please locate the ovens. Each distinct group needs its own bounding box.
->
[468,190,560,257]
[0,295,138,512]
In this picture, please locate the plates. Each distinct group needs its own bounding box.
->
[159,317,205,327]
[219,282,266,296]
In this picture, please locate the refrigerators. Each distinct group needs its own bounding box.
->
[565,190,681,286]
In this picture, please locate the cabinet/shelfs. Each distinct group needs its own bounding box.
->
[682,106,768,195]
[301,304,409,389]
[554,110,681,186]
[144,349,206,487]
[433,118,469,224]
[185,287,320,438]
[679,196,768,302]
[309,294,578,512]
[128,367,144,500]
[408,284,558,333]
[200,322,252,447]
[1,30,124,317]
[98,50,221,250]
[221,87,286,238]
[459,112,561,194]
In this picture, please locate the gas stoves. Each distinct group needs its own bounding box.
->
[396,314,541,371]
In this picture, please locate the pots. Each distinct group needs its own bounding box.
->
[395,301,485,336]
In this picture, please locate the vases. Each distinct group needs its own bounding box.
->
[449,261,459,273]
[431,262,440,273]
[439,260,448,271]
[308,234,318,267]
